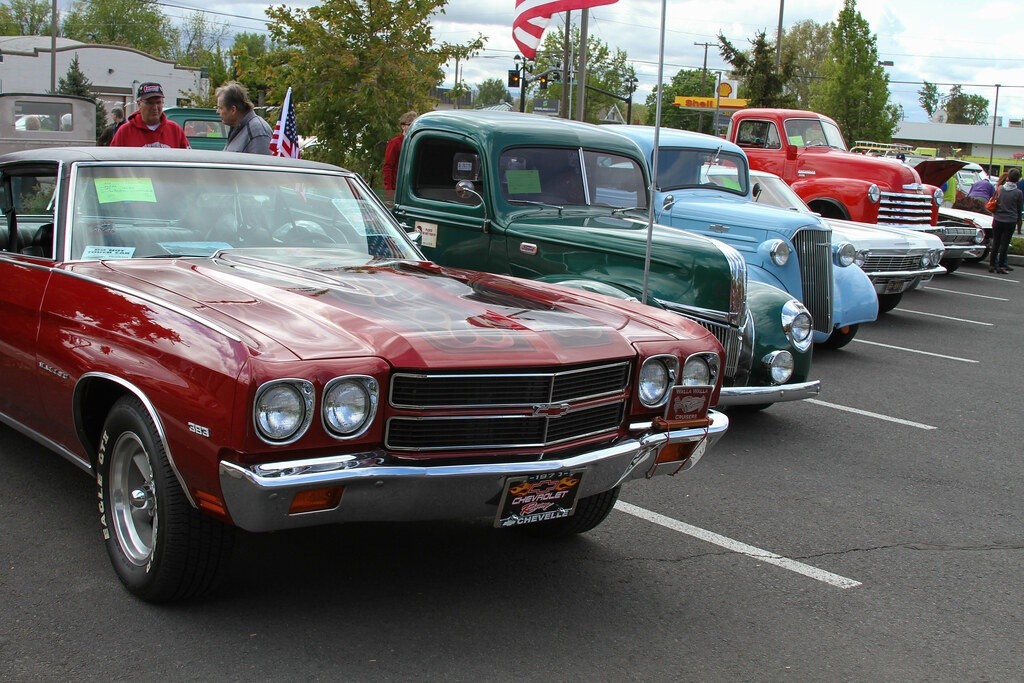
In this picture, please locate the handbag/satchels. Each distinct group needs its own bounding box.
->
[985,188,999,213]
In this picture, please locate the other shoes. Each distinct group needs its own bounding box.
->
[1000,264,1013,271]
[990,267,1009,274]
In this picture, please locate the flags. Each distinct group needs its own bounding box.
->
[512,0,619,61]
[269,88,301,159]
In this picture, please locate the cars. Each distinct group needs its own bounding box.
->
[591,121,881,351]
[698,163,947,313]
[727,109,988,275]
[0,147,733,603]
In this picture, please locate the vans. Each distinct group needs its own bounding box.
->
[907,158,995,198]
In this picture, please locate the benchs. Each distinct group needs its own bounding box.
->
[1,222,203,258]
[416,188,568,202]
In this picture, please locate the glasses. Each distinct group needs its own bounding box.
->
[402,122,411,126]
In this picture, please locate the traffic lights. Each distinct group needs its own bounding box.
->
[553,62,561,80]
[540,75,546,88]
[509,71,519,87]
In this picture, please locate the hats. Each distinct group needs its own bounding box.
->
[136,82,165,100]
[896,153,905,159]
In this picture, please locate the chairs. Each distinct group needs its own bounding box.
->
[21,221,91,262]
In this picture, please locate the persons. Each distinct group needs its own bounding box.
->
[111,82,191,149]
[988,168,1024,276]
[112,107,127,146]
[216,84,272,155]
[25,116,40,131]
[938,176,957,209]
[384,111,417,204]
[967,178,994,208]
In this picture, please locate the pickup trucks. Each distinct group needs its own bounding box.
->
[267,107,821,416]
[160,105,234,151]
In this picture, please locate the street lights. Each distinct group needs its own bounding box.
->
[715,71,722,136]
[515,55,535,112]
